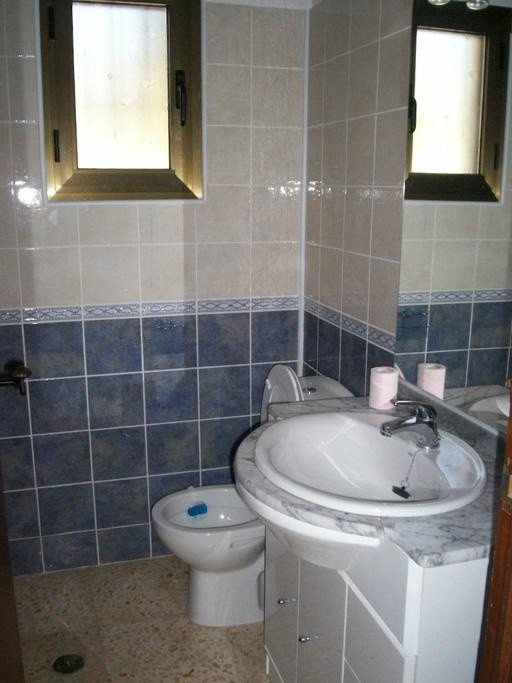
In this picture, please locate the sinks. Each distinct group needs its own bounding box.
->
[469,395,511,438]
[254,411,488,519]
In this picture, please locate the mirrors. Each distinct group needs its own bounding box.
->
[395,0,510,442]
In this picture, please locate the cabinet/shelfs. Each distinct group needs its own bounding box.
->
[261,528,490,682]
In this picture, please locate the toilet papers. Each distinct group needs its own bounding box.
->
[417,362,446,400]
[369,366,399,409]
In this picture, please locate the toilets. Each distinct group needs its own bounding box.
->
[151,363,362,627]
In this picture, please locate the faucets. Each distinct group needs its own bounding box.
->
[380,400,438,448]
